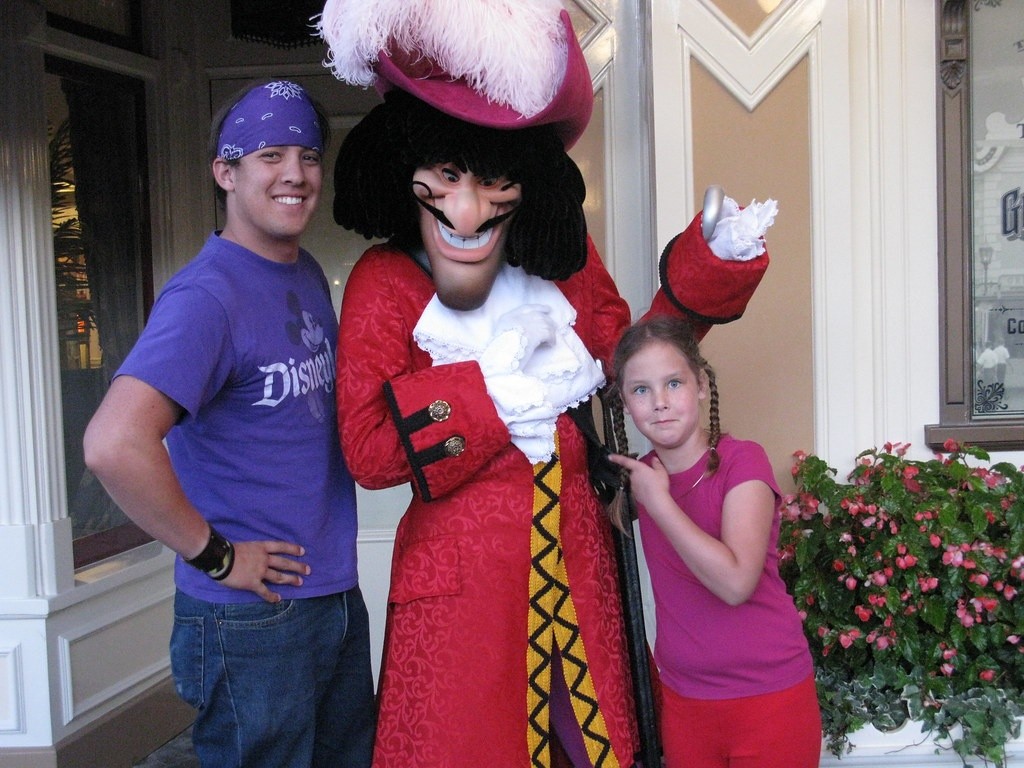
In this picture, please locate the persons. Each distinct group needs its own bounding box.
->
[608,319,821,768]
[320,0,778,768]
[977,339,1014,389]
[83,74,375,768]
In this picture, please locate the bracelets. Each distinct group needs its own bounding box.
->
[183,525,235,581]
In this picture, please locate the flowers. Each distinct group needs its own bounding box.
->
[777,436,1024,750]
